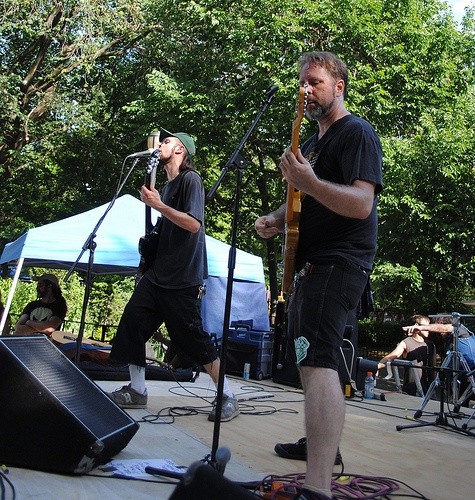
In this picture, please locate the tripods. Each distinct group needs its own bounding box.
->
[145,88,277,488]
[396,371,475,437]
[414,313,474,418]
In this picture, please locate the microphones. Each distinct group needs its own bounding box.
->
[129,147,155,158]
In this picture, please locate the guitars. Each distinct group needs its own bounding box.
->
[51,330,176,371]
[281,83,309,294]
[135,149,161,286]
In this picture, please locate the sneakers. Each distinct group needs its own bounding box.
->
[106,383,148,408]
[292,490,337,500]
[274,438,342,465]
[208,393,240,421]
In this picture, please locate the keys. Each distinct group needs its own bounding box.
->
[198,286,202,299]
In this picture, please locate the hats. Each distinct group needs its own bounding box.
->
[159,127,196,156]
[32,273,58,287]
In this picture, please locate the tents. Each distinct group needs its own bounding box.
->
[0,194,270,339]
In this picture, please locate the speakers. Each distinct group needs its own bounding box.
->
[0,333,140,476]
[273,293,359,396]
[221,327,274,378]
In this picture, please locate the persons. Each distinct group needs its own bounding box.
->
[255,51,385,500]
[0,290,12,336]
[104,125,241,423]
[14,273,67,337]
[374,313,475,408]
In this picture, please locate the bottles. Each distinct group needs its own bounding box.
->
[244,363,250,380]
[364,372,375,399]
